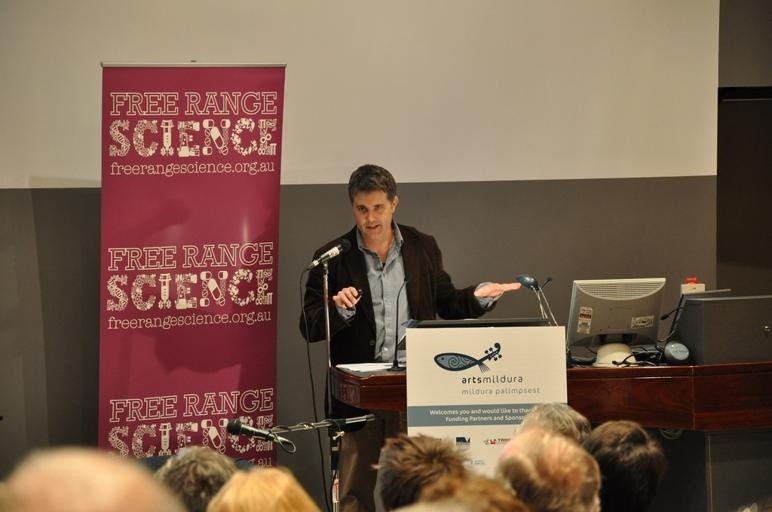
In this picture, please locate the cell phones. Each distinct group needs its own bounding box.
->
[346,287,367,304]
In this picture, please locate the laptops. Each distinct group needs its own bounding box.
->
[655,288,733,348]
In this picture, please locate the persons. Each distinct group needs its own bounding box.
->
[2,444,318,511]
[299,164,522,512]
[375,400,666,512]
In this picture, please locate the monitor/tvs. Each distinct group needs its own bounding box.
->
[566,277,667,369]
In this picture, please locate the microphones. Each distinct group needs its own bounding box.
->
[306,237,350,275]
[660,305,683,321]
[387,278,412,373]
[224,416,288,448]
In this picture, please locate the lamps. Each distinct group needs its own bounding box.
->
[516,274,572,369]
